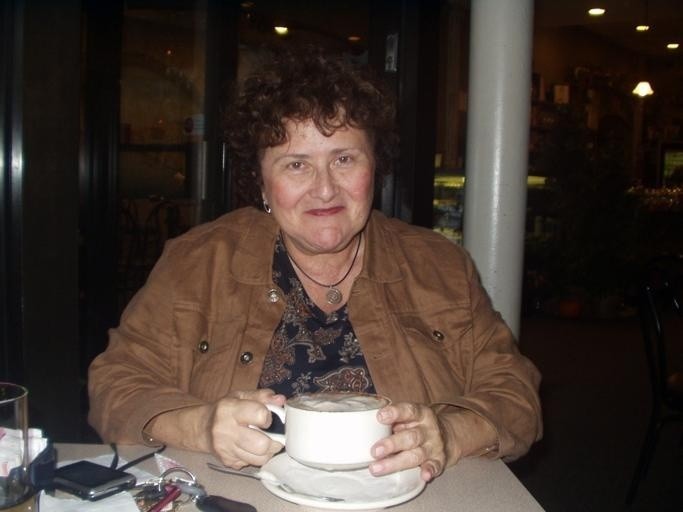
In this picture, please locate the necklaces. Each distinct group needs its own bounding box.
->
[279,228,362,304]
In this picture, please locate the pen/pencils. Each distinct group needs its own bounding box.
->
[147,485,181,511]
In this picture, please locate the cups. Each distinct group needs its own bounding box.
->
[0,382,35,512]
[248,391,392,473]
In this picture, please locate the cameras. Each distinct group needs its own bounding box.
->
[53,461,136,499]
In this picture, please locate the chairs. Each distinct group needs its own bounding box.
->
[624,261,682,505]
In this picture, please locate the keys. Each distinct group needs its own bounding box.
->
[131,467,257,512]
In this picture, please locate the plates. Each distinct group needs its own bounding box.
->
[259,451,427,511]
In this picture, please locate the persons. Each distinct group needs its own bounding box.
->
[87,50,543,480]
